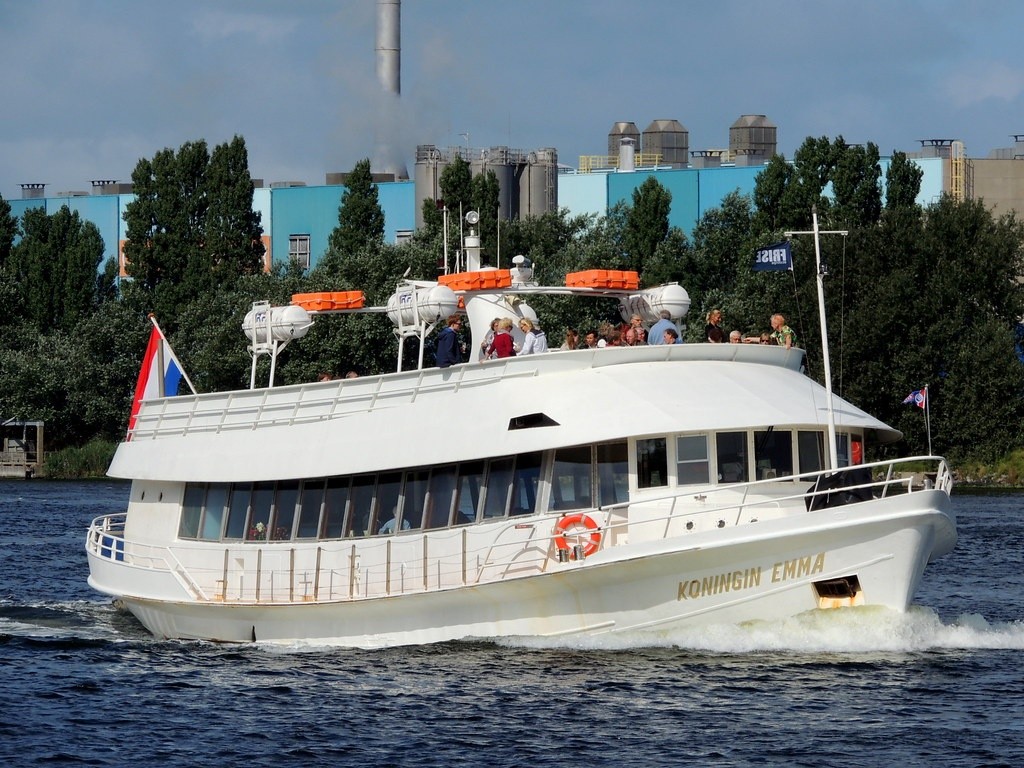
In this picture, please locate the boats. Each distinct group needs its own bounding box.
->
[83,199,958,651]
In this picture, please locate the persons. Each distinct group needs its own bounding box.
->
[435,314,462,368]
[730,331,742,343]
[663,329,683,344]
[705,310,723,344]
[347,371,359,378]
[560,329,579,352]
[480,318,548,364]
[759,333,772,345]
[378,506,410,534]
[648,310,682,344]
[319,372,331,381]
[579,313,648,350]
[743,314,797,350]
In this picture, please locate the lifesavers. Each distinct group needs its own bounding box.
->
[554,513,602,560]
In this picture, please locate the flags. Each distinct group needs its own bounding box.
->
[902,389,925,408]
[753,240,791,270]
[127,326,183,442]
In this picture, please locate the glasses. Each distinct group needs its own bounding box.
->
[521,325,526,329]
[454,323,461,326]
[509,325,513,330]
[761,339,770,342]
[632,316,643,322]
